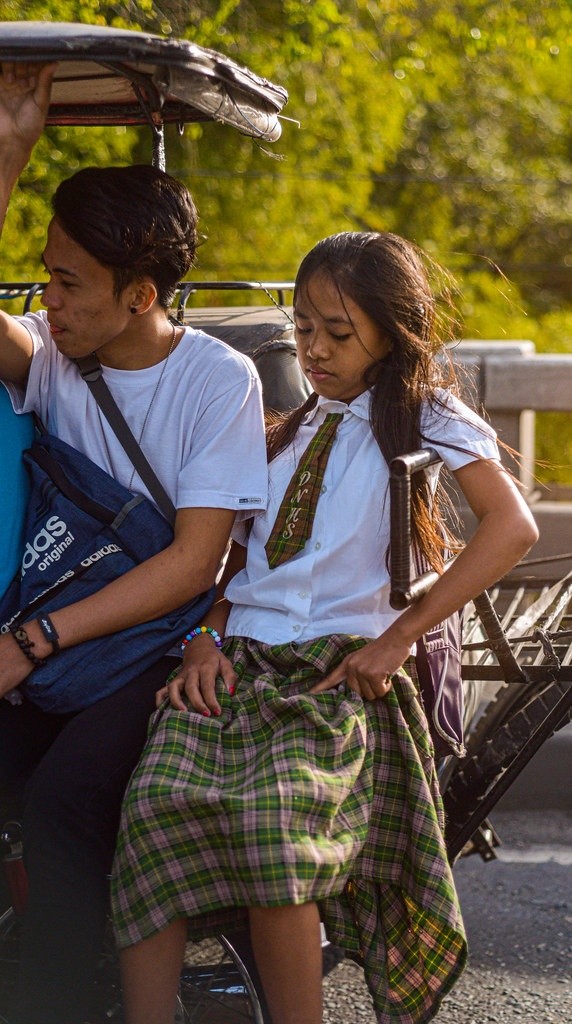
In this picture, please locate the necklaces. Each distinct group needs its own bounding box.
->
[98,319,176,492]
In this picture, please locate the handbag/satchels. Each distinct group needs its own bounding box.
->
[1,425,223,716]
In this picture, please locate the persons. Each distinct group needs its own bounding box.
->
[0,60,270,1024]
[114,234,540,1023]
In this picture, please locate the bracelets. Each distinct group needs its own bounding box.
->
[181,626,223,655]
[9,625,47,666]
[36,612,60,653]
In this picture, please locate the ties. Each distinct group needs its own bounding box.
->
[264,413,344,570]
